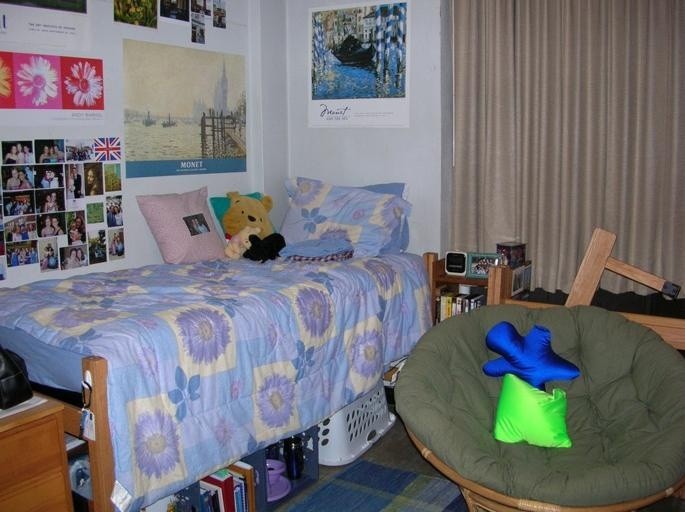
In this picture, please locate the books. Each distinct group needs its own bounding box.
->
[195,461,255,512]
[432,285,486,322]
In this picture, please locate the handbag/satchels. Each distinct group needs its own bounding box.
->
[0,343,34,410]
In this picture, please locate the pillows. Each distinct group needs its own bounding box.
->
[136,186,228,266]
[209,191,276,256]
[493,373,572,448]
[482,321,580,391]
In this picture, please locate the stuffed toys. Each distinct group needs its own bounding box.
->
[224,225,262,260]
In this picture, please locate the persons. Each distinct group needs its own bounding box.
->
[107,205,115,227]
[111,232,116,257]
[39,217,54,237]
[24,146,33,164]
[17,247,20,255]
[54,145,65,162]
[44,192,59,212]
[114,206,122,226]
[18,170,32,189]
[41,170,50,188]
[87,169,101,195]
[52,218,64,235]
[116,235,124,256]
[24,247,31,263]
[189,218,200,234]
[16,144,24,164]
[4,168,20,189]
[21,222,28,238]
[194,218,208,232]
[75,216,86,242]
[45,195,51,202]
[68,219,82,245]
[5,146,17,164]
[28,221,37,238]
[77,248,88,269]
[48,243,56,267]
[20,247,27,264]
[40,146,51,163]
[73,167,83,199]
[49,147,57,163]
[62,248,79,269]
[13,224,23,241]
[66,171,75,199]
[50,170,59,188]
[41,246,48,270]
[10,248,19,265]
[31,246,37,263]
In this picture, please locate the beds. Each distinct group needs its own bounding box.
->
[1,252,429,512]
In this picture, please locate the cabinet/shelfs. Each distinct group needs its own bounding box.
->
[429,253,495,326]
[0,395,75,512]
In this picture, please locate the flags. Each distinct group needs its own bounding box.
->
[94,137,122,161]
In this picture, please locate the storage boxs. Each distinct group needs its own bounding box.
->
[318,382,396,467]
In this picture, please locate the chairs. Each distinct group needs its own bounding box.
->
[394,304,684,512]
